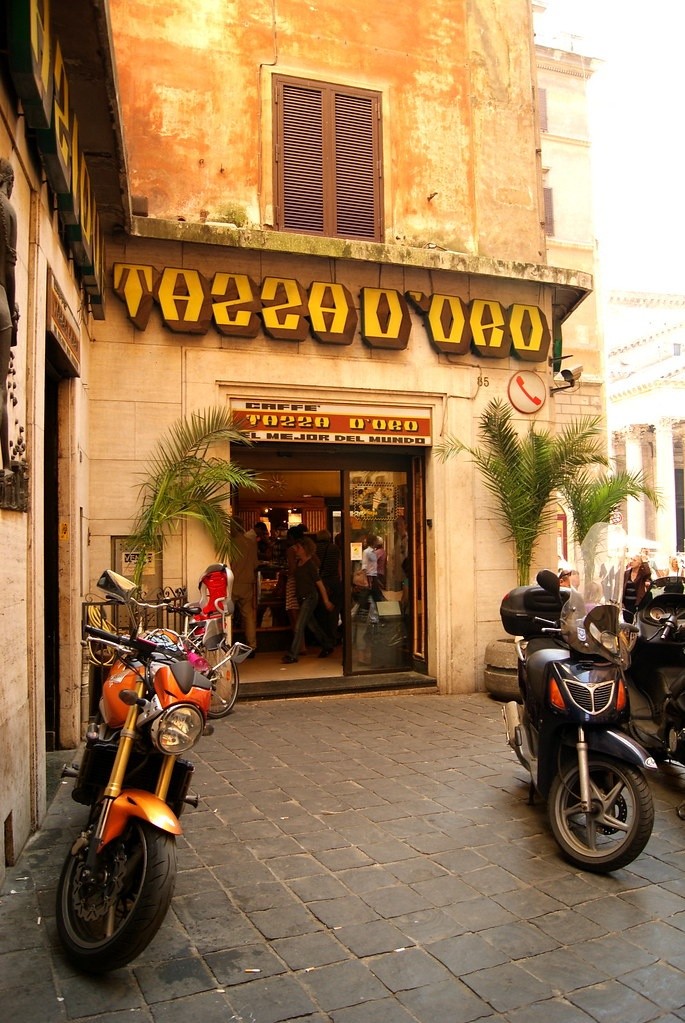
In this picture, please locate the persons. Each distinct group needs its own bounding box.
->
[231,515,343,664]
[352,532,387,623]
[401,556,410,617]
[558,547,685,659]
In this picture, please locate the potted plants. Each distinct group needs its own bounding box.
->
[96,405,267,707]
[434,396,601,704]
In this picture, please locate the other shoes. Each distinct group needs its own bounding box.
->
[318,648,333,658]
[282,656,296,662]
[241,651,255,658]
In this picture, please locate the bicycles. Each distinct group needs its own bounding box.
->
[102,595,239,719]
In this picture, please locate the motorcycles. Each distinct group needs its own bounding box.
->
[56,570,256,974]
[607,575,684,821]
[498,570,658,874]
